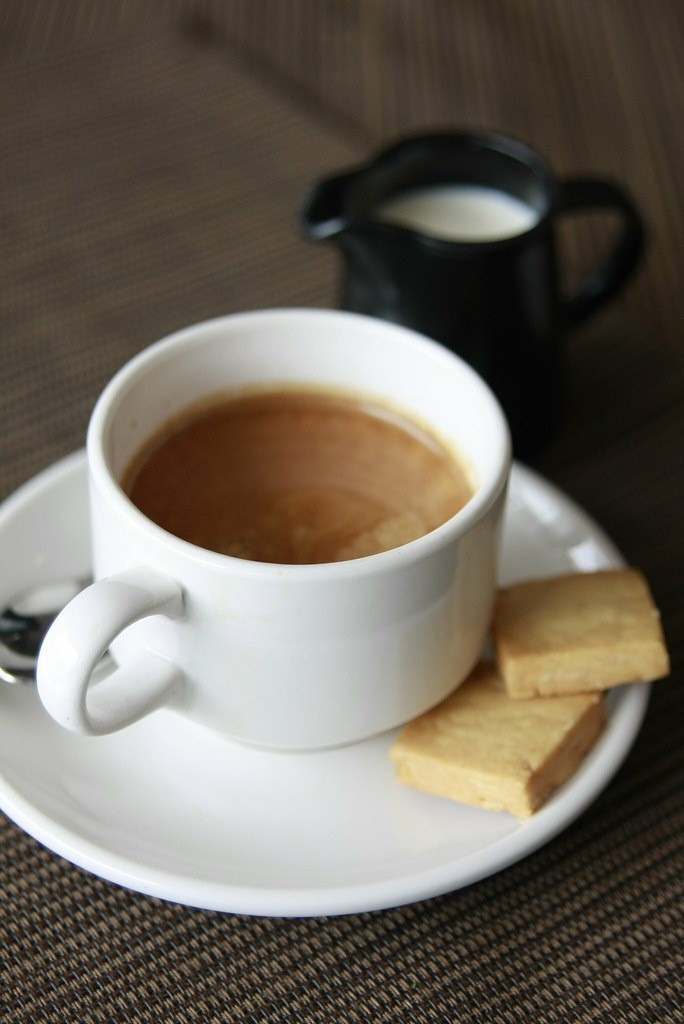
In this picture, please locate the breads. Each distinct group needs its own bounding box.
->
[392,661,606,817]
[491,567,670,700]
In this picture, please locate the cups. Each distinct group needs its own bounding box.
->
[35,308,509,751]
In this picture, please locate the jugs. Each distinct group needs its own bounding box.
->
[295,120,650,416]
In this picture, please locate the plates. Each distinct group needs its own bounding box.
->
[0,452,652,918]
